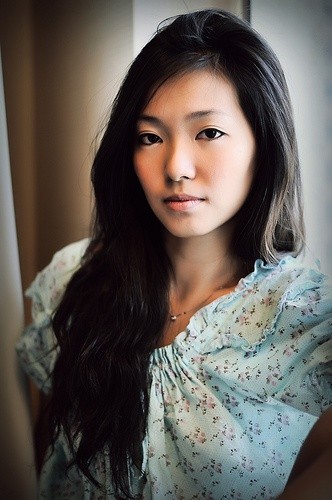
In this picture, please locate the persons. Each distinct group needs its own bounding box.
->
[12,9,332,498]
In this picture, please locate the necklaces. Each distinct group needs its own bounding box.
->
[165,284,223,323]
